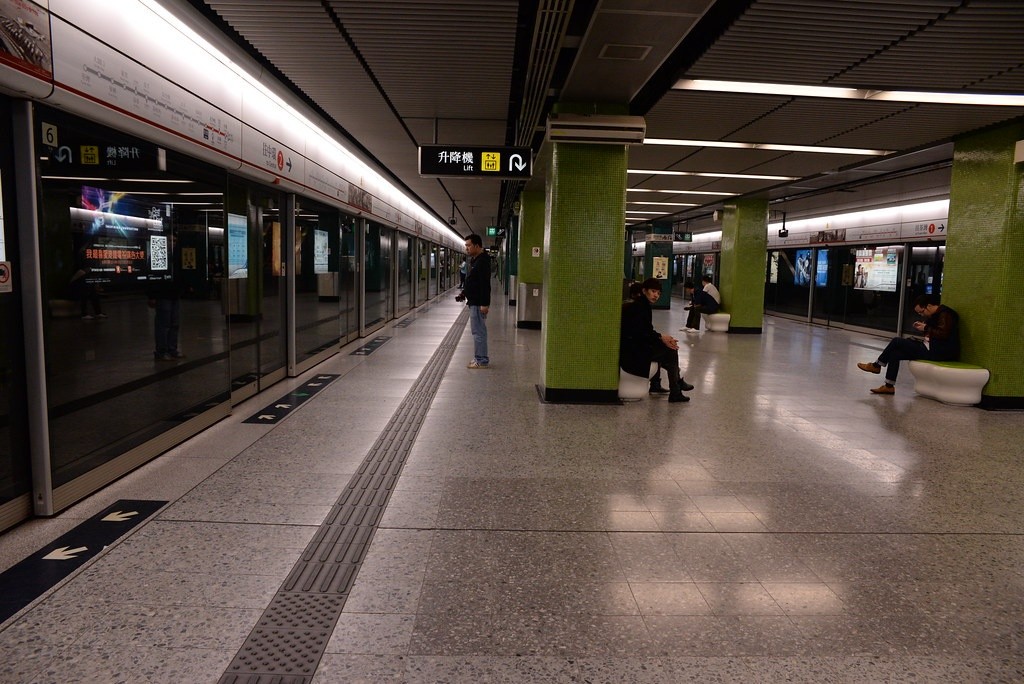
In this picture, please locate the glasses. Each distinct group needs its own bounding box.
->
[918,308,925,316]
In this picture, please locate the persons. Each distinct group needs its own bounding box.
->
[679,275,721,333]
[857,294,961,395]
[492,259,498,278]
[136,229,186,361]
[458,257,467,289]
[797,251,812,287]
[73,233,109,319]
[459,234,491,369]
[855,264,868,288]
[619,278,694,403]
[918,265,925,286]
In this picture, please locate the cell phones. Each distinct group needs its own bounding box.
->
[455,296,465,301]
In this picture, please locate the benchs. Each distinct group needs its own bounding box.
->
[701,312,731,332]
[618,361,659,402]
[907,360,990,406]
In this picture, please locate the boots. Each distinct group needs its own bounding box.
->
[669,385,690,402]
[678,377,694,391]
[649,379,669,393]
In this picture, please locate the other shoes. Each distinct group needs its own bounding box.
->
[870,385,895,394]
[687,328,699,332]
[858,363,880,374]
[153,351,185,361]
[471,358,477,364]
[468,362,489,369]
[679,326,690,330]
